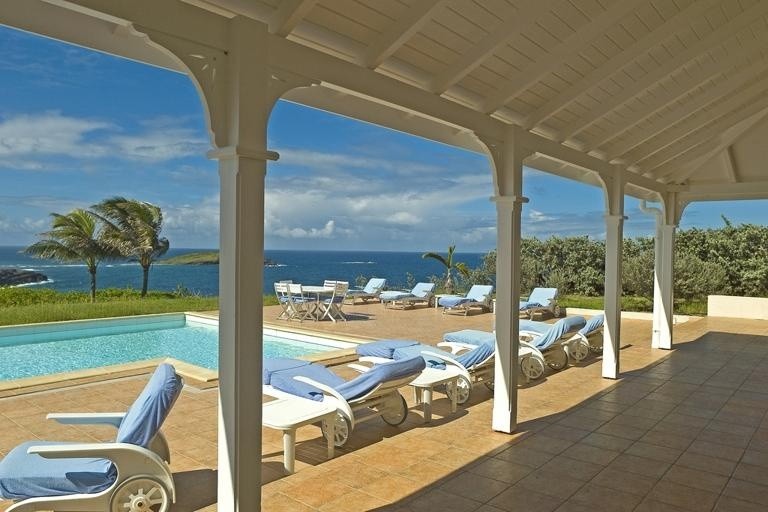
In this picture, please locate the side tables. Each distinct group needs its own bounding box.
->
[263,396,338,477]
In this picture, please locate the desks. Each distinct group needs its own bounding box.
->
[405,368,459,423]
[517,348,533,384]
[561,334,582,364]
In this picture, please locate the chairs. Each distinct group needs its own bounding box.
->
[353,340,495,404]
[0,362,184,512]
[345,278,560,322]
[265,355,425,444]
[492,317,605,361]
[273,280,349,323]
[443,316,588,384]
